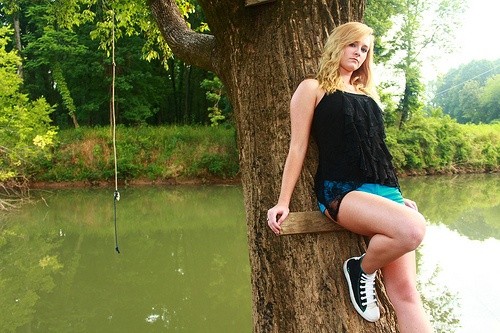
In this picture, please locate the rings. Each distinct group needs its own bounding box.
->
[266,217,269,222]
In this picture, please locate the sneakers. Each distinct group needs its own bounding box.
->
[343,253,381,322]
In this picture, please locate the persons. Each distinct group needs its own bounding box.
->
[265,21,435,333]
